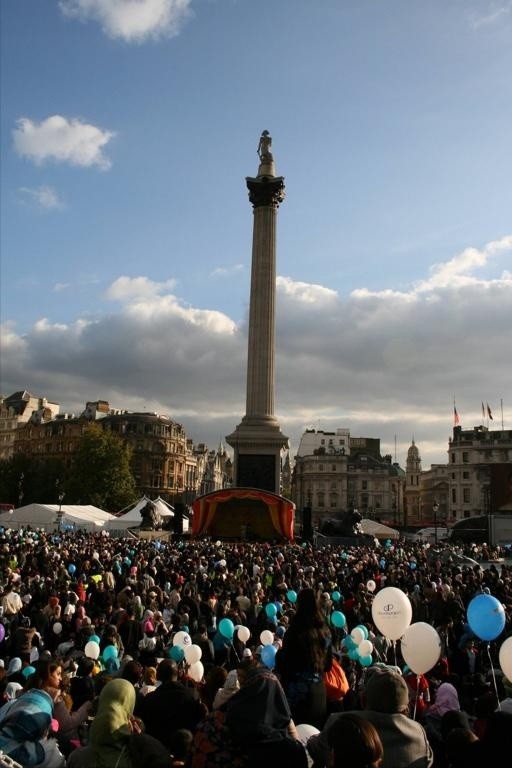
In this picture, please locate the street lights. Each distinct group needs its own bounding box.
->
[57,490,65,531]
[432,500,441,545]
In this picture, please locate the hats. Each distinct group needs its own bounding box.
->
[364,671,410,713]
